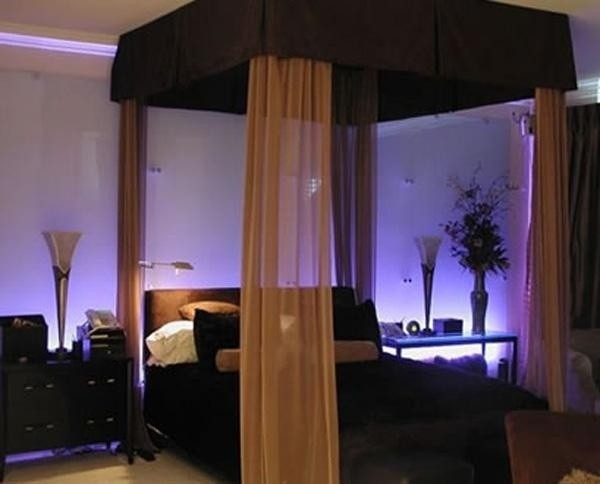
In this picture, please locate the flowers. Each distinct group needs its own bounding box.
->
[436,158,522,283]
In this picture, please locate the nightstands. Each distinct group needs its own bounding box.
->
[0,350,136,482]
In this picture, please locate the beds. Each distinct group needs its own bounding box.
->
[145,281,551,484]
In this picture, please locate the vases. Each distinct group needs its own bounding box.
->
[470,271,489,338]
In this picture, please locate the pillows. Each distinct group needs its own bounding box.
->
[145,298,387,398]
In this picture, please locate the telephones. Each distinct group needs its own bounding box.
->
[85,310,124,330]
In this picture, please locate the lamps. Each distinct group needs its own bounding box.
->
[413,234,444,335]
[138,260,194,271]
[40,227,82,354]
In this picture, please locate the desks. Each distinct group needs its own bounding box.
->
[383,329,520,385]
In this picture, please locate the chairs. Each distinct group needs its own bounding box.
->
[565,327,600,398]
[506,407,598,484]
